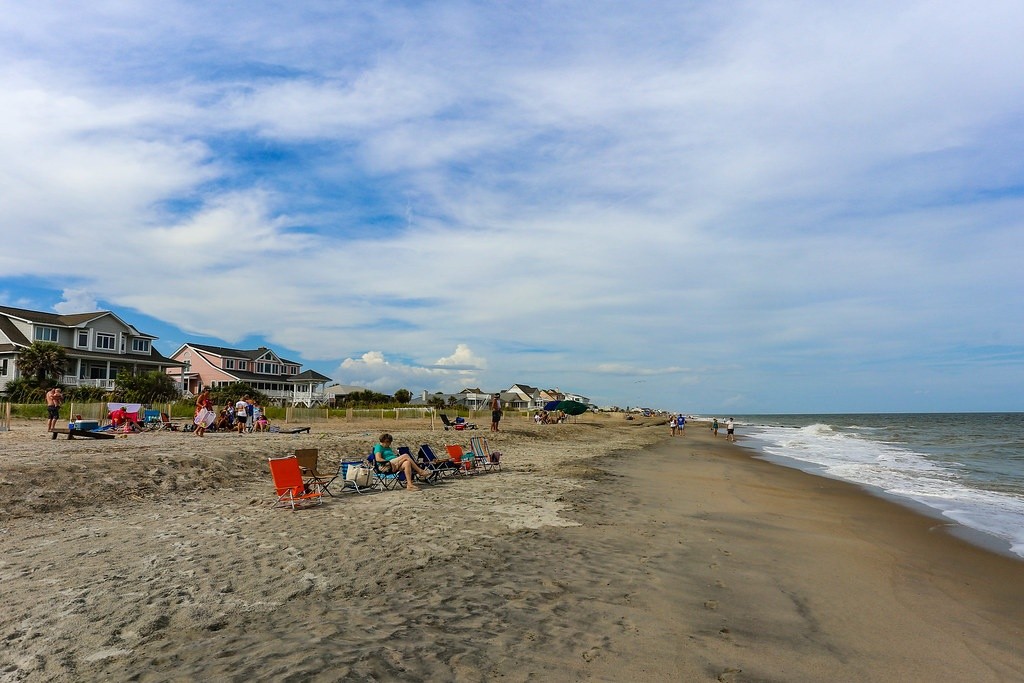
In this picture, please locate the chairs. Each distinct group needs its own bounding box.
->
[268,436,502,510]
[439,414,456,428]
[158,412,181,432]
[560,414,568,423]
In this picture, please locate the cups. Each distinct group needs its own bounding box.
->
[433,459,436,462]
[419,458,423,463]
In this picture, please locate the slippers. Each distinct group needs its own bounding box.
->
[425,469,435,479]
[407,485,421,490]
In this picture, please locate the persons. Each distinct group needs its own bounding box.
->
[194,387,213,437]
[218,394,267,433]
[535,410,565,424]
[491,393,503,432]
[713,419,718,437]
[670,416,676,436]
[726,418,734,441]
[374,434,433,491]
[108,407,141,430]
[45,387,63,432]
[677,414,685,436]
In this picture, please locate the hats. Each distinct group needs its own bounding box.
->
[120,406,127,409]
[205,386,212,391]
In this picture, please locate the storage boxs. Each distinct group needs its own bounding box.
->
[454,424,466,429]
[68,423,74,429]
[194,408,217,428]
[74,420,98,430]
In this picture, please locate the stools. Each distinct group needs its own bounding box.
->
[147,422,158,431]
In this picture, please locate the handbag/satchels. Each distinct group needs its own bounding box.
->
[194,407,216,428]
[346,463,373,486]
